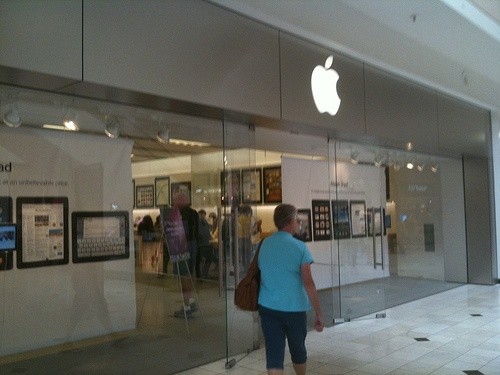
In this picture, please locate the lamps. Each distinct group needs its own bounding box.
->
[374,154,439,174]
[3,106,23,128]
[63,114,79,132]
[105,118,121,140]
[350,147,359,164]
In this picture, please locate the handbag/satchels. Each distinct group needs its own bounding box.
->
[235,236,265,311]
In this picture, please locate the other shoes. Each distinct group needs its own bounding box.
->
[174,308,191,318]
[190,302,200,312]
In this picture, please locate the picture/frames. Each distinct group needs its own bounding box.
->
[135,177,192,209]
[221,165,282,207]
[293,199,387,242]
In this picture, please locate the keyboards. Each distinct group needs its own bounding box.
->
[77,236,126,258]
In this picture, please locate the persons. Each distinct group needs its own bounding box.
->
[172,192,200,304]
[138,214,170,272]
[253,203,324,375]
[197,206,261,279]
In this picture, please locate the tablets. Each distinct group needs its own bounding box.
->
[0,196,129,271]
[130,177,191,209]
[220,166,282,207]
[0,222,17,251]
[293,199,386,243]
